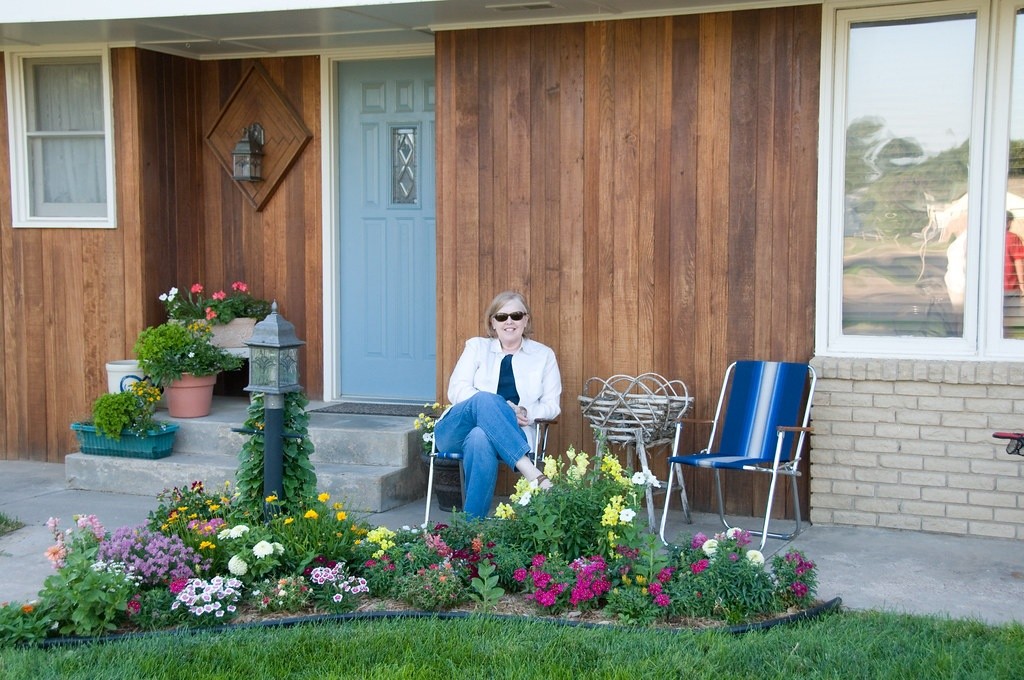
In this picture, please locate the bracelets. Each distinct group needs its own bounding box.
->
[1018,282,1024,284]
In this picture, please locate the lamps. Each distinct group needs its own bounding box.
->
[230,120,264,184]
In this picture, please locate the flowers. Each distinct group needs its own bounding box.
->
[127,326,243,385]
[89,382,169,444]
[414,402,450,457]
[158,281,272,327]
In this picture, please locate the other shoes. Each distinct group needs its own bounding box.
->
[536,476,553,490]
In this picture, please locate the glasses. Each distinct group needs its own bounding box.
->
[492,311,528,322]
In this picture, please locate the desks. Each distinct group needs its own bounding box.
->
[578,372,692,535]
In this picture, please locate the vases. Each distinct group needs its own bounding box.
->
[71,421,179,460]
[162,370,217,419]
[420,454,462,512]
[168,314,256,349]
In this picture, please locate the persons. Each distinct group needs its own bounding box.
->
[1004,210,1024,292]
[433,290,562,525]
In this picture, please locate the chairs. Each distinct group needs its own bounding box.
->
[659,359,816,552]
[424,404,557,529]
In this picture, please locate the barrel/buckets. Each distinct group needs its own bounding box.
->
[106,359,155,412]
